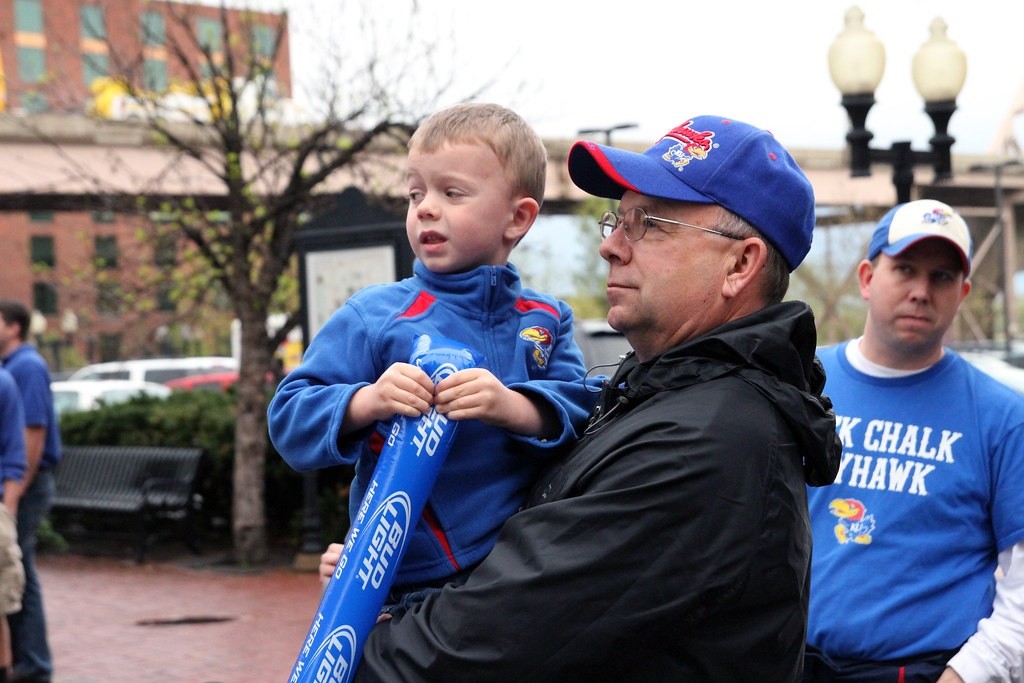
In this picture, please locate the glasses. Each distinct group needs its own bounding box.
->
[597,212,749,243]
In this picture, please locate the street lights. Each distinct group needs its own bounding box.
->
[969,161,1020,358]
[29,308,79,375]
[579,124,639,212]
[828,5,967,205]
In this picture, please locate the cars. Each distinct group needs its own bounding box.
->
[164,371,277,394]
[68,357,242,382]
[952,341,1024,396]
[50,380,172,420]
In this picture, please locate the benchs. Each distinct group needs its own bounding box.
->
[47,447,213,564]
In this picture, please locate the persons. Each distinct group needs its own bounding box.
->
[0,364,29,683]
[806,198,1024,683]
[267,104,624,597]
[318,117,842,683]
[0,300,63,683]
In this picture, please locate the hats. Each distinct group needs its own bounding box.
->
[568,116,814,269]
[864,198,973,281]
